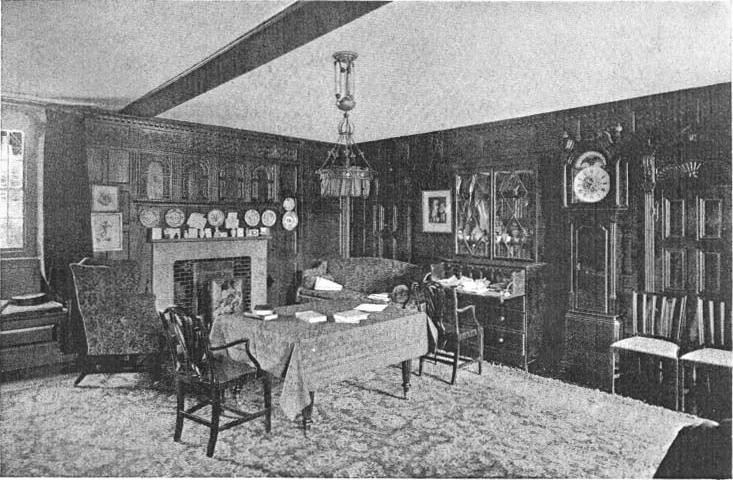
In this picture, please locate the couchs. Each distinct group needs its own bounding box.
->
[293,255,426,307]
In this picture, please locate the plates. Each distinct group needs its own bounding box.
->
[282,198,299,231]
[139,208,184,227]
[244,209,276,226]
[208,209,224,227]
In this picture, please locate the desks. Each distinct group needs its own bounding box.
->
[209,298,430,439]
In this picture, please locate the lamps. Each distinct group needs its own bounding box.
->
[315,50,379,200]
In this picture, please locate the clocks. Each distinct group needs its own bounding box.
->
[571,166,612,204]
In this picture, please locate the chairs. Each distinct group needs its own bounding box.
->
[606,285,731,415]
[417,285,487,385]
[158,305,272,458]
[70,256,162,388]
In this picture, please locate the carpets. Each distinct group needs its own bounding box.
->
[0,350,715,477]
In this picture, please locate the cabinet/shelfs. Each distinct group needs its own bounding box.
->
[427,258,550,373]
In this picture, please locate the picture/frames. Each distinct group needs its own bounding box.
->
[420,189,454,235]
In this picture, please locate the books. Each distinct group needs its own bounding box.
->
[295,310,327,323]
[332,308,369,324]
[243,304,278,322]
